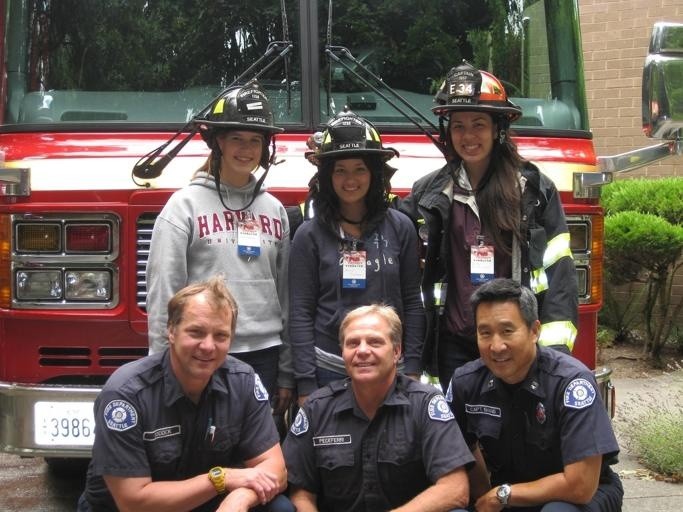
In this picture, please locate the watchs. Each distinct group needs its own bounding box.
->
[208,466,227,493]
[495,482,510,505]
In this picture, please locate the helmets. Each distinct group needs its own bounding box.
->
[311,105,394,160]
[430,65,523,117]
[194,78,285,136]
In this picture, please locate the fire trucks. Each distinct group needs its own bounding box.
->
[0,0,683,460]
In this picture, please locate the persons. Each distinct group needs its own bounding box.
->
[398,70,580,391]
[145,86,291,413]
[285,106,425,407]
[79,281,287,512]
[445,278,624,512]
[281,305,476,512]
